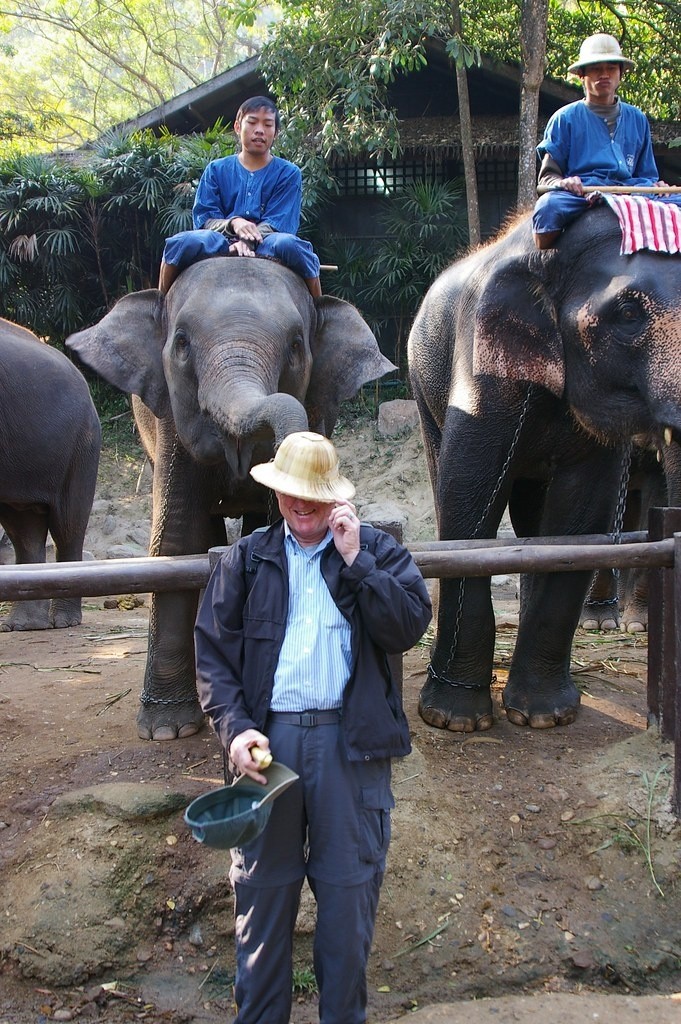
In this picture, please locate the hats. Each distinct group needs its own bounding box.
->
[567,34,638,75]
[250,431,356,504]
[184,761,300,849]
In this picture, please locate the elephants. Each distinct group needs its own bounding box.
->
[0,317,105,632]
[66,254,398,740]
[408,183,681,733]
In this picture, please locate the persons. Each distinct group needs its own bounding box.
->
[531,33,681,251]
[195,432,432,1024]
[158,96,323,297]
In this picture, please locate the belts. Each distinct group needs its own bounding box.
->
[269,712,340,726]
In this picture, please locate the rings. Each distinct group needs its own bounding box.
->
[247,250,250,251]
[348,512,354,519]
